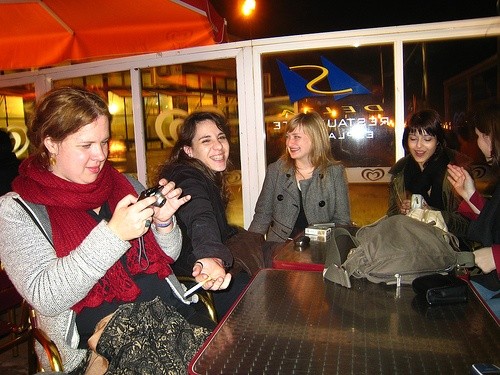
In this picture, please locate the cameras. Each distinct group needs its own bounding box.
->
[411,194,431,211]
[137,185,166,208]
[306,227,331,237]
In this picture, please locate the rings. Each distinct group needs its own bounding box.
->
[193,261,204,271]
[144,218,151,227]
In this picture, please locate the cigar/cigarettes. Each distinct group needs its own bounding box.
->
[183,276,212,298]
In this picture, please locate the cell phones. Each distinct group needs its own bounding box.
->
[472,363,500,375]
[296,236,310,246]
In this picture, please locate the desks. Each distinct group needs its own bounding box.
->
[272,225,361,272]
[187,269,500,375]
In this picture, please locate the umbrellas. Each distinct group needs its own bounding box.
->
[0,0,228,71]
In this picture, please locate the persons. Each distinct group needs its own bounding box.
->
[0,85,218,375]
[155,111,252,324]
[387,109,500,320]
[247,111,350,243]
[0,127,22,197]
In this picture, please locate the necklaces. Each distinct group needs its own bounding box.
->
[297,169,305,179]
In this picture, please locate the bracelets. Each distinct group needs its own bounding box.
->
[214,257,223,268]
[152,217,174,227]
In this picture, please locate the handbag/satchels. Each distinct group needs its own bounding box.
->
[323,214,476,288]
[67,297,211,375]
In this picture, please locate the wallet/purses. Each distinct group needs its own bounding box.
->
[412,274,467,304]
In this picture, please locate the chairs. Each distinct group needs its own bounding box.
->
[0,270,217,375]
